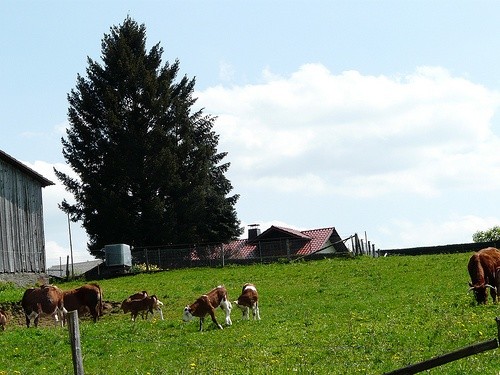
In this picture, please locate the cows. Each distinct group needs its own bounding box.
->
[22,281,103,328]
[467,246,499,306]
[182,285,233,332]
[233,283,261,322]
[118,290,165,322]
